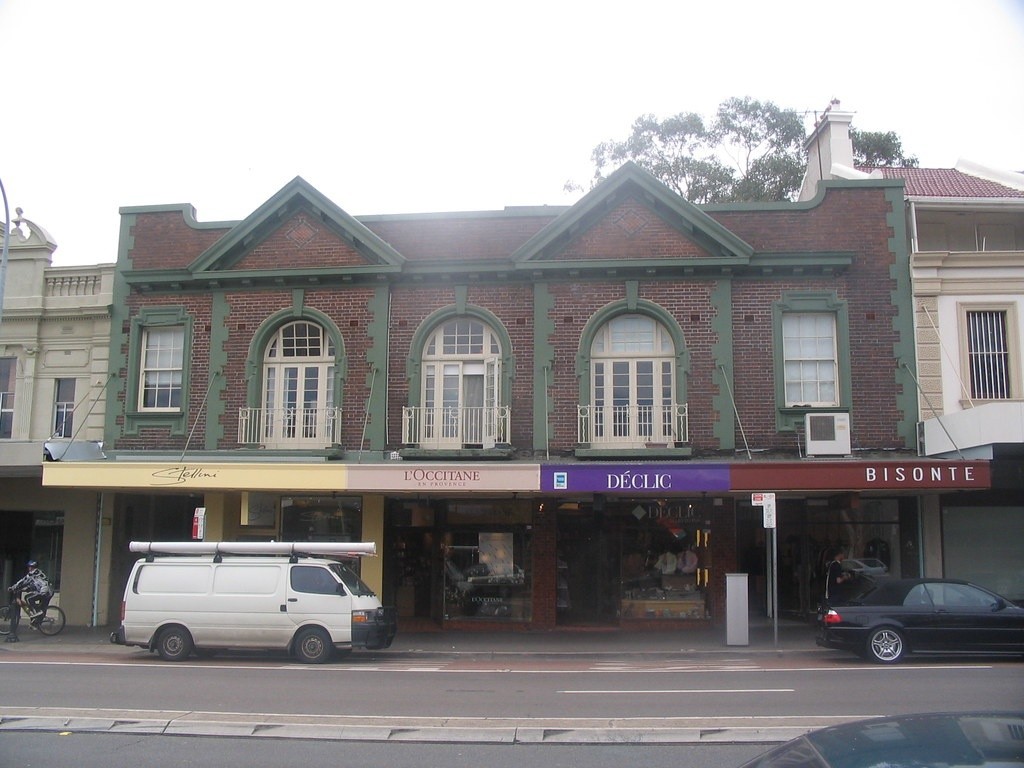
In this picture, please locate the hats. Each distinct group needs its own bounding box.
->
[26,560,40,568]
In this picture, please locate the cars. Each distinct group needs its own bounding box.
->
[449,562,524,604]
[815,575,1024,665]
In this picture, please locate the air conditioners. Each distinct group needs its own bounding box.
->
[803,412,851,455]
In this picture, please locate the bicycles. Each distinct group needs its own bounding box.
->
[0,585,65,637]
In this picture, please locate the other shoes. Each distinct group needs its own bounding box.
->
[31,625,37,631]
[30,610,43,618]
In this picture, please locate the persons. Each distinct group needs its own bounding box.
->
[655,553,677,575]
[676,550,698,572]
[816,547,852,607]
[6,560,54,631]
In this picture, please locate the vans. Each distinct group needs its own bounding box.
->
[110,557,396,663]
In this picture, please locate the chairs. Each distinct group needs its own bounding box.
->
[921,589,935,608]
